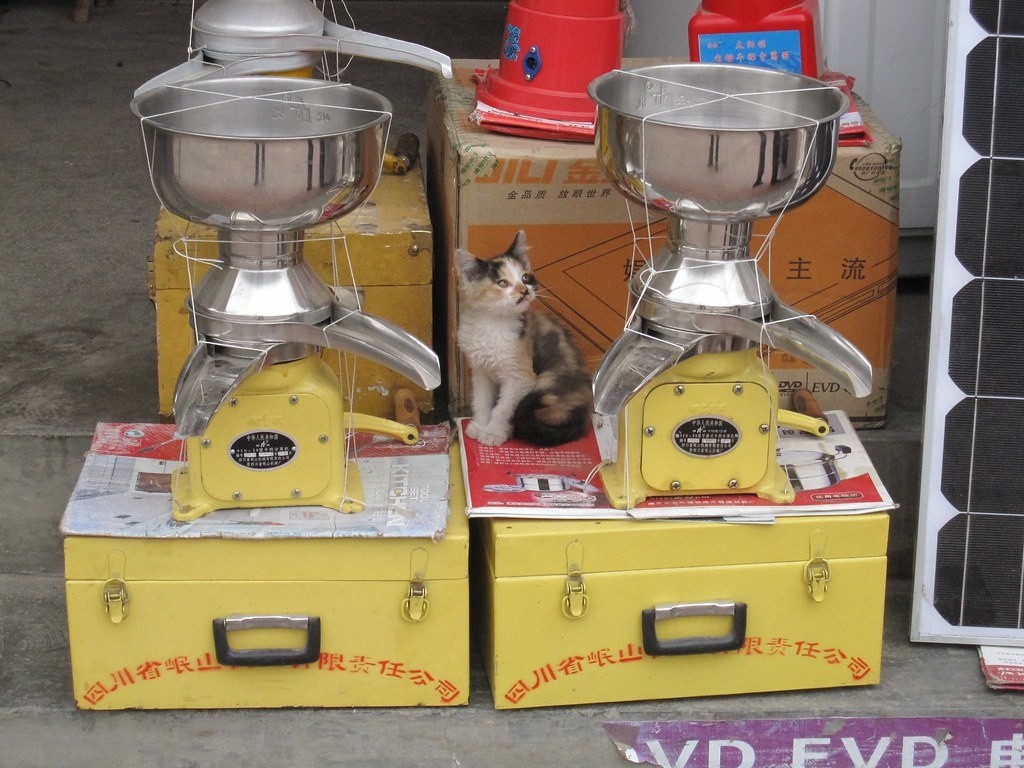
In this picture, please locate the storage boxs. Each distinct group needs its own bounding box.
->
[478,513,889,710]
[147,153,435,424]
[426,57,901,430]
[64,437,468,710]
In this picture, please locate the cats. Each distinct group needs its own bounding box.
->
[453,229,594,448]
